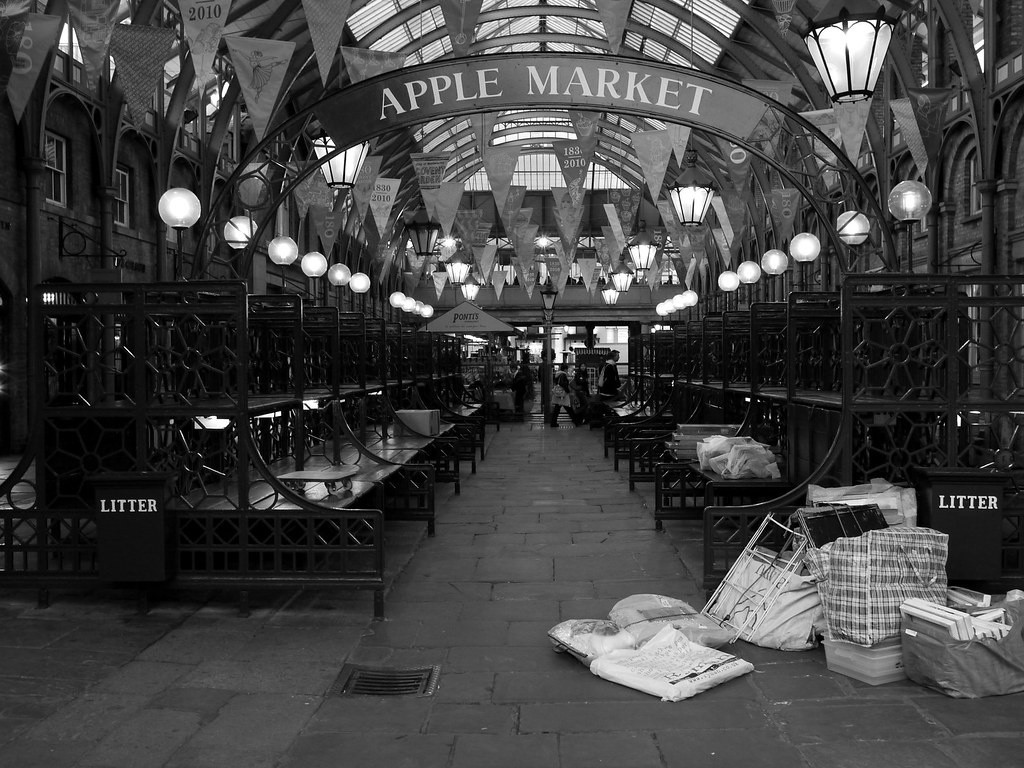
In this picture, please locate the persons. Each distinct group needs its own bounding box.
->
[574,361,592,396]
[597,350,621,401]
[506,362,527,413]
[549,364,582,428]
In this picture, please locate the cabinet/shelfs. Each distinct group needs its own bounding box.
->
[1,277,531,618]
[575,268,1023,596]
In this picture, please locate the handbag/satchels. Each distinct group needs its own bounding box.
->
[568,372,583,392]
[551,373,571,408]
[826,525,950,646]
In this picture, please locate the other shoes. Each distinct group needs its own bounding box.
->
[576,418,585,427]
[550,423,560,427]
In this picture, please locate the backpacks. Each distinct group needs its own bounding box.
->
[596,364,612,387]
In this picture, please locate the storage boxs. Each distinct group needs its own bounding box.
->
[821,630,908,685]
[393,407,440,438]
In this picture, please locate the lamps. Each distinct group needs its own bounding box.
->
[888,181,931,273]
[673,293,686,320]
[421,304,433,331]
[159,187,201,279]
[540,281,558,310]
[268,236,299,293]
[761,249,788,302]
[224,217,258,270]
[655,303,668,331]
[405,122,442,256]
[328,263,351,312]
[666,0,719,227]
[401,297,415,324]
[790,233,821,290]
[601,278,621,306]
[609,254,635,292]
[664,298,676,330]
[717,270,740,313]
[301,252,328,306]
[682,290,699,320]
[349,271,371,311]
[389,291,406,324]
[310,34,372,188]
[460,268,481,303]
[624,118,661,271]
[736,261,761,307]
[413,301,424,331]
[800,1,896,104]
[444,241,473,286]
[837,211,870,270]
